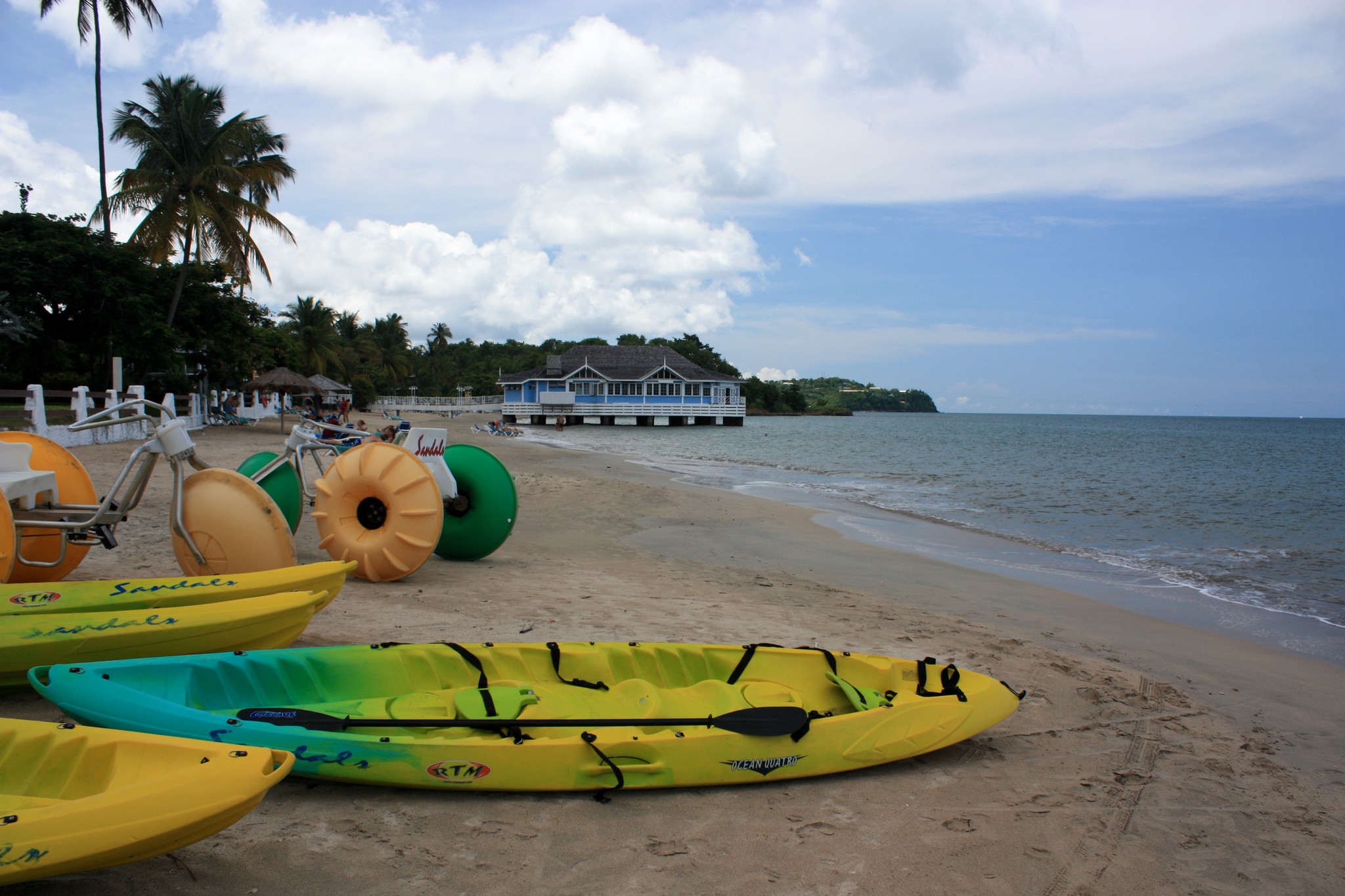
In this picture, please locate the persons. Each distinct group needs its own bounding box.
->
[215,394,395,450]
[487,420,525,436]
[555,415,566,431]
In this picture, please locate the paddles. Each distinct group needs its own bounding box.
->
[235,707,809,736]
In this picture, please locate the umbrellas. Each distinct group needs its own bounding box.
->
[240,367,323,435]
[306,374,353,416]
[294,389,338,397]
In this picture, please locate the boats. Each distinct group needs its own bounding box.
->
[0,557,364,688]
[0,715,296,890]
[29,635,1030,804]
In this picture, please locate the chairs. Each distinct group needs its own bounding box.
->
[394,421,413,436]
[315,424,402,456]
[274,405,340,423]
[207,406,259,427]
[471,422,525,437]
[381,408,405,421]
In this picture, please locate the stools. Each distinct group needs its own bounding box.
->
[0,438,62,512]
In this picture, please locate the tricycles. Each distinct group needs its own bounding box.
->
[235,402,518,585]
[0,396,299,583]
